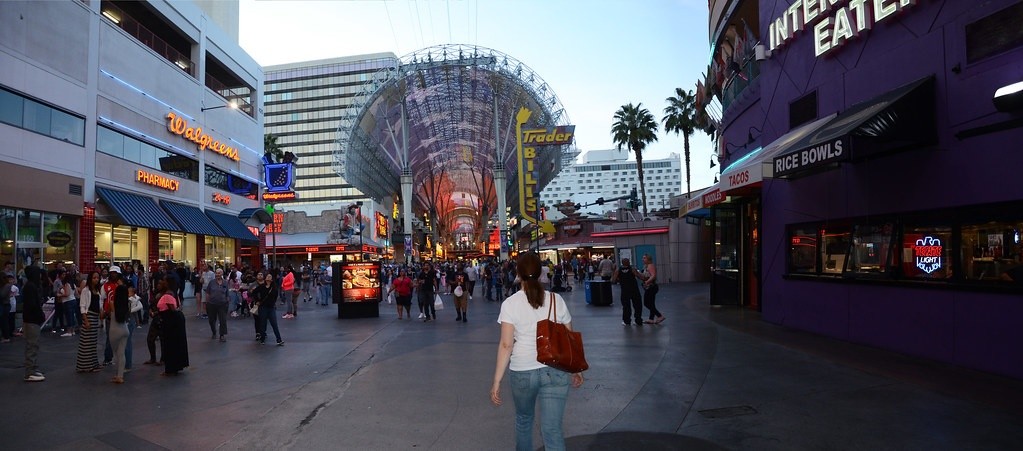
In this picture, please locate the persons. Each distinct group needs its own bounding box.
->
[598,254,613,281]
[206,268,231,343]
[22,264,47,381]
[344,208,365,239]
[559,254,618,286]
[974,243,994,257]
[0,256,555,383]
[612,253,666,327]
[490,250,584,451]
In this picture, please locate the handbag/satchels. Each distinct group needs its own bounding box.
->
[454,282,463,297]
[537,292,589,373]
[434,294,444,311]
[250,305,259,315]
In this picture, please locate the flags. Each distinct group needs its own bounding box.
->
[694,23,758,139]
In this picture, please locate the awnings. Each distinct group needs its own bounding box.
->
[678,183,743,221]
[773,74,936,181]
[718,113,837,194]
[205,208,259,242]
[593,245,615,249]
[557,247,577,250]
[159,199,226,237]
[239,206,272,233]
[96,187,181,231]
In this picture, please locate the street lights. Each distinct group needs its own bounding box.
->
[357,201,363,262]
[384,215,388,266]
[533,191,539,257]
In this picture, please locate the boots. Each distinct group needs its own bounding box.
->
[456,310,462,321]
[462,312,468,323]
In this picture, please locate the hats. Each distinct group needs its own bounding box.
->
[108,265,122,274]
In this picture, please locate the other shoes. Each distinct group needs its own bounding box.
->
[633,321,641,327]
[212,332,216,339]
[102,362,115,365]
[407,311,410,320]
[219,334,226,343]
[425,315,431,321]
[277,341,284,346]
[398,313,402,319]
[622,321,630,326]
[432,313,436,319]
[282,313,297,318]
[261,340,264,345]
[230,311,238,317]
[419,313,425,319]
[153,360,164,366]
[110,376,125,383]
[60,332,76,336]
[23,371,45,381]
[143,360,155,364]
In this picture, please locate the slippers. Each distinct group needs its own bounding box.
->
[655,316,666,325]
[642,319,654,324]
